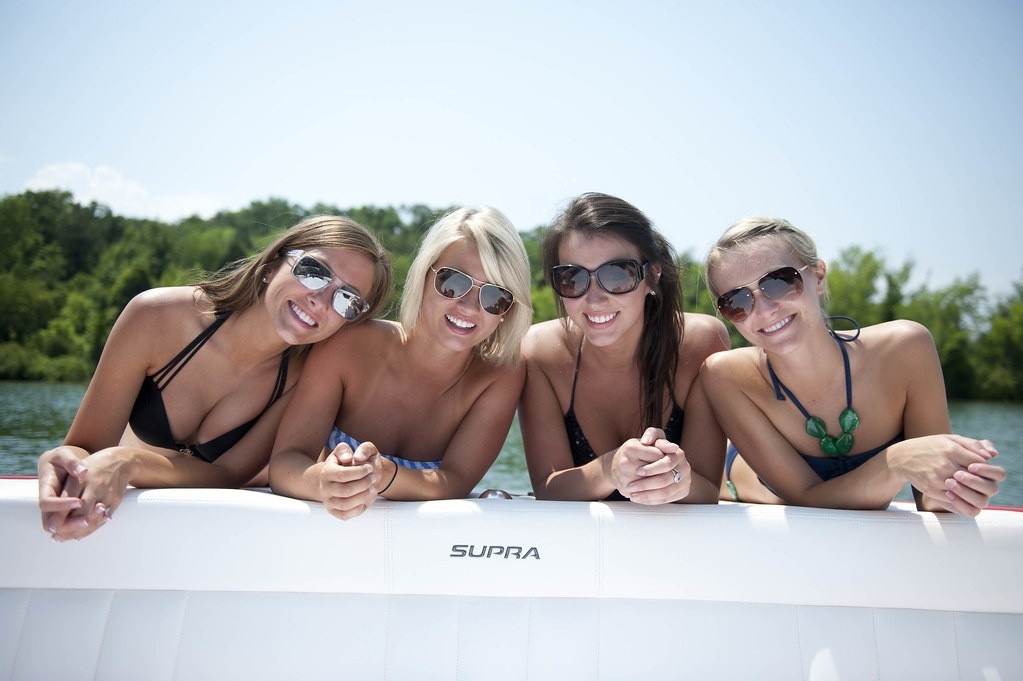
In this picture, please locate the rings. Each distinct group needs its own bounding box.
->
[103,505,113,517]
[671,468,682,483]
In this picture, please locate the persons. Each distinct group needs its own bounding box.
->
[516,191,733,506]
[268,201,535,521]
[698,214,1006,517]
[37,215,393,543]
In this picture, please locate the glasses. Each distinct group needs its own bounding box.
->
[552,258,650,298]
[429,265,517,317]
[284,249,371,323]
[717,263,812,323]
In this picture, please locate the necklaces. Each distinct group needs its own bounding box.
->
[765,331,861,456]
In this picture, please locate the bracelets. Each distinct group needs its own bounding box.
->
[378,459,398,495]
